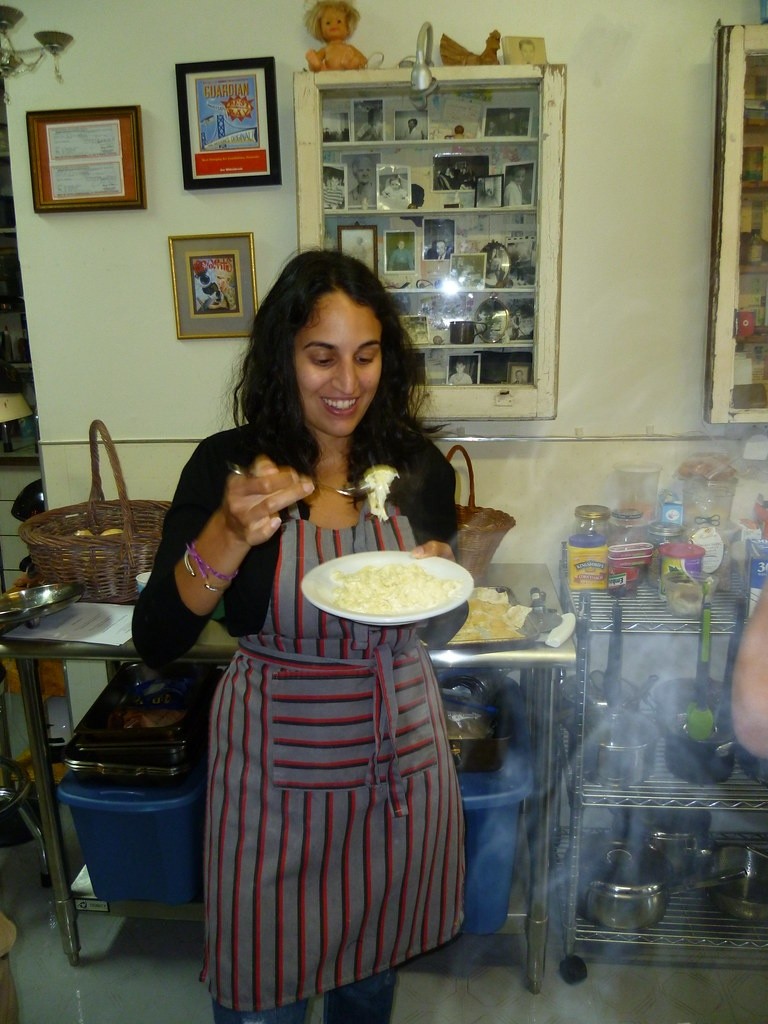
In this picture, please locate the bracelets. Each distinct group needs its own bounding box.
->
[182,549,227,592]
[185,540,238,581]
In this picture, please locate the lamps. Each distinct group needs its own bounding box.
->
[0,5,73,105]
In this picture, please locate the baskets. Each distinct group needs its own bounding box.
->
[17,419,172,604]
[445,445,516,577]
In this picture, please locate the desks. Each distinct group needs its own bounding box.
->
[0,564,579,994]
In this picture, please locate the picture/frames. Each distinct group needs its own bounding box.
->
[27,105,144,213]
[168,232,258,340]
[174,56,282,191]
[338,175,536,387]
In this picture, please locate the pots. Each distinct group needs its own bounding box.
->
[557,672,768,933]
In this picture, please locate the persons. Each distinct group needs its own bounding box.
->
[356,108,383,141]
[400,118,424,140]
[348,155,377,205]
[433,240,451,259]
[350,237,368,262]
[486,247,504,279]
[503,165,525,206]
[449,360,473,385]
[132,246,468,1024]
[387,238,414,270]
[729,585,768,759]
[302,0,369,73]
[382,177,407,199]
[518,38,535,64]
[513,369,528,385]
[477,302,496,340]
[323,173,343,210]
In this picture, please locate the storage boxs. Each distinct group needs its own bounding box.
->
[61,663,542,936]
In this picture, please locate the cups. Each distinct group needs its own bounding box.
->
[681,477,737,529]
[135,572,151,593]
[738,312,756,337]
[449,320,488,344]
[615,459,662,525]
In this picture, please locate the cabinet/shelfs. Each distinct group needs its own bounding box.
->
[707,22,768,427]
[290,64,570,421]
[554,561,768,985]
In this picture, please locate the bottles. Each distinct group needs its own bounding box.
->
[566,505,706,620]
[747,229,763,266]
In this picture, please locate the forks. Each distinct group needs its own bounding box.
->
[229,463,375,498]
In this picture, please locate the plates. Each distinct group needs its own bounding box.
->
[300,550,474,625]
